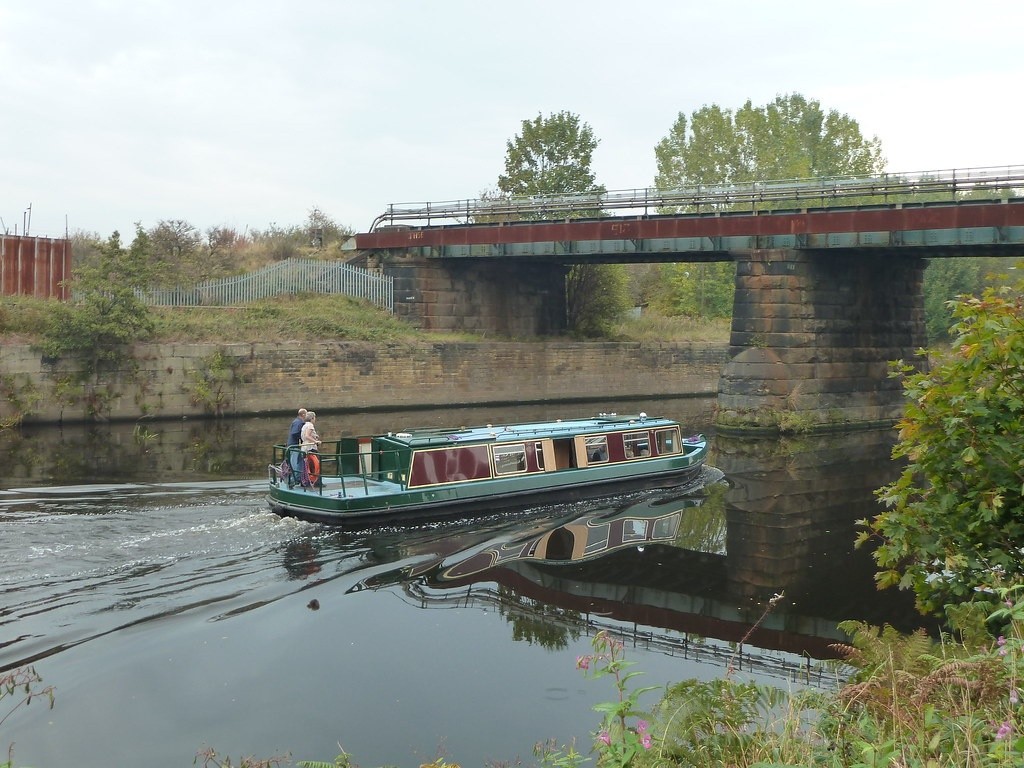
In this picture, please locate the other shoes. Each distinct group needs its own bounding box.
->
[316,482,327,487]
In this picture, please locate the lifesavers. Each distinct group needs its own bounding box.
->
[306,454,320,482]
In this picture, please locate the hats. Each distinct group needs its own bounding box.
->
[304,411,316,421]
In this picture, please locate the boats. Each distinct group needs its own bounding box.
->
[265,412,708,533]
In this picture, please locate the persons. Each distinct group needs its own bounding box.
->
[300,412,327,487]
[288,408,308,484]
[593,445,608,461]
